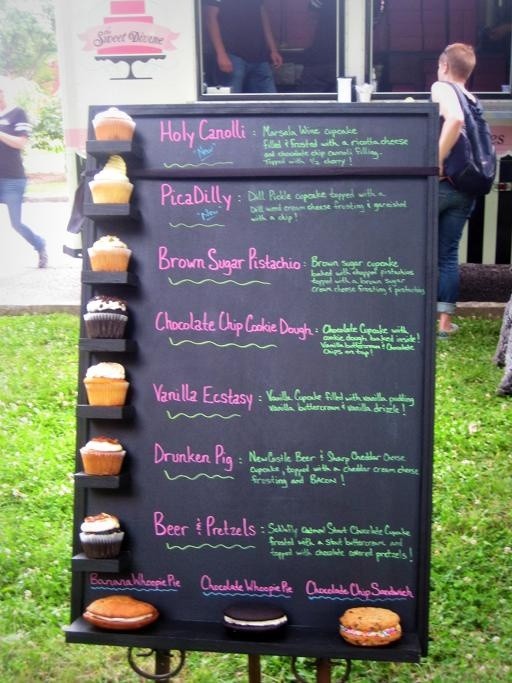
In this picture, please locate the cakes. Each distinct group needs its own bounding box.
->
[83,293,129,340]
[87,232,132,273]
[90,154,134,205]
[96,1,163,56]
[93,108,133,141]
[79,435,126,476]
[78,511,126,559]
[338,606,404,648]
[83,360,129,408]
[82,596,160,628]
[217,603,291,635]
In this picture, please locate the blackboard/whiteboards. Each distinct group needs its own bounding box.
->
[65,102,439,662]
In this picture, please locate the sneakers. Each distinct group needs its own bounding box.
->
[438,324,458,339]
[37,238,47,267]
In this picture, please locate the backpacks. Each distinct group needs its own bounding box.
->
[443,81,496,194]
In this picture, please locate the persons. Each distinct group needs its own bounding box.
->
[0,86,49,268]
[291,0,384,92]
[475,0,512,85]
[203,1,284,93]
[431,41,479,337]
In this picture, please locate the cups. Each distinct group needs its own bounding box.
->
[335,75,372,104]
